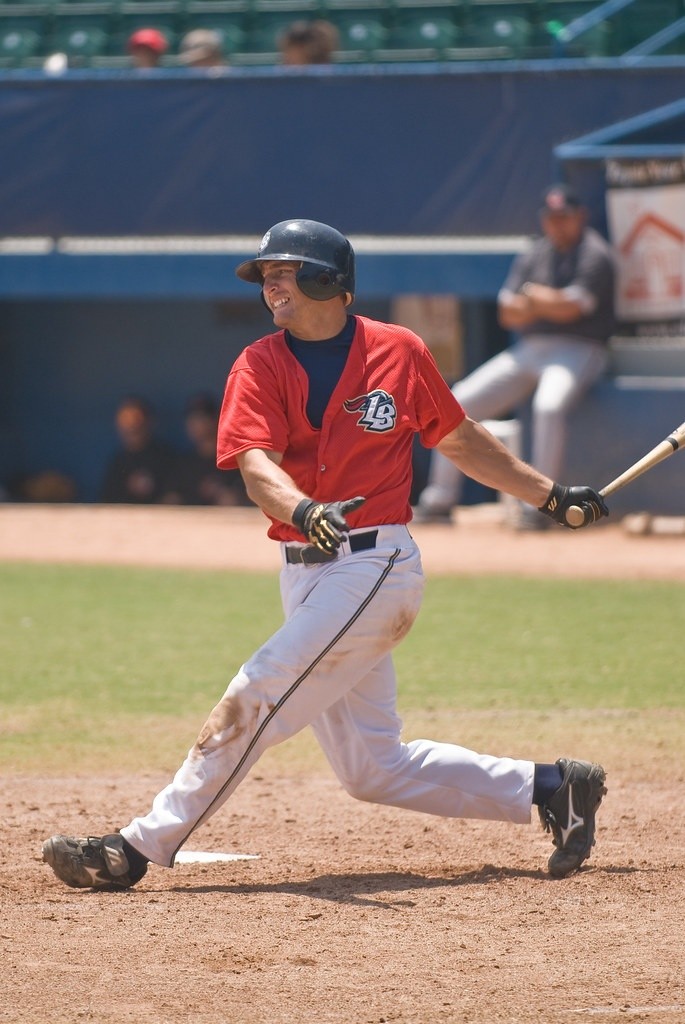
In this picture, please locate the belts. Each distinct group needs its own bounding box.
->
[286,530,380,563]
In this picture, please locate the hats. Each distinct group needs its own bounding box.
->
[535,184,584,217]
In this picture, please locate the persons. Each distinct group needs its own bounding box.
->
[126,29,165,69]
[411,185,617,532]
[180,30,229,67]
[90,396,247,506]
[278,20,338,65]
[41,219,608,891]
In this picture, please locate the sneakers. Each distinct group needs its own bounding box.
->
[539,759,608,877]
[44,831,148,891]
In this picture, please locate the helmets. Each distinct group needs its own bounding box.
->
[236,219,356,315]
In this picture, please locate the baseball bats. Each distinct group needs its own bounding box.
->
[562,420,685,529]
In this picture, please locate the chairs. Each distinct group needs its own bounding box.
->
[0,0,685,69]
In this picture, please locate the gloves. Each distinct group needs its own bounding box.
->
[539,483,610,529]
[303,496,366,557]
